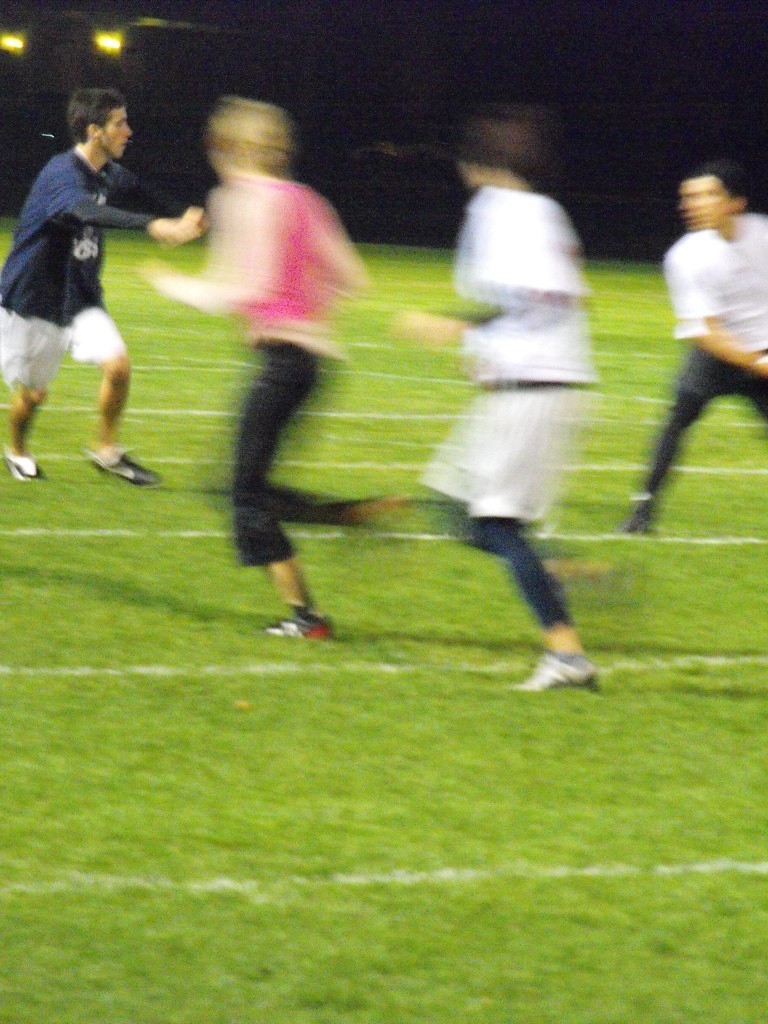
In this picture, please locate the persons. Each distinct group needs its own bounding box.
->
[0,90,212,489]
[395,110,602,692]
[629,157,768,539]
[147,92,411,643]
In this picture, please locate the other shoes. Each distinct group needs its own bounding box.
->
[618,498,655,534]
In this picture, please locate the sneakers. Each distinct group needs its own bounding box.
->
[514,651,595,692]
[91,448,165,487]
[4,451,47,481]
[264,615,337,640]
